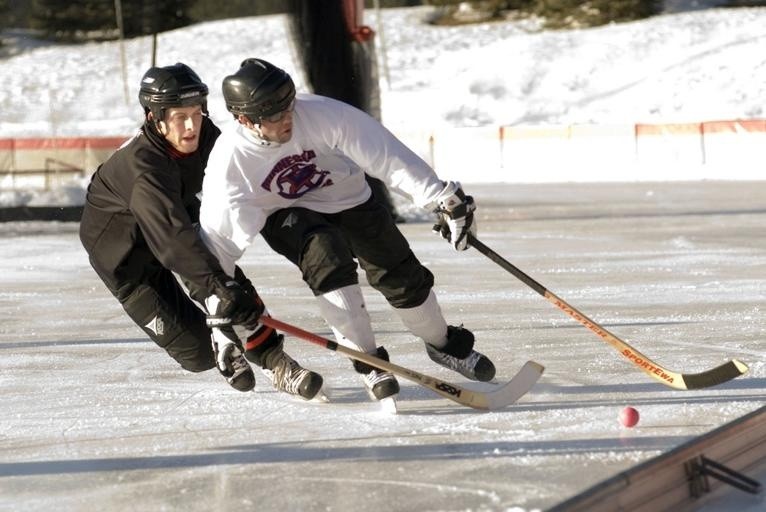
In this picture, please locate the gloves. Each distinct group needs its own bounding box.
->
[422,179,477,252]
[198,272,271,378]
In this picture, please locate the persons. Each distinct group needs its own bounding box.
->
[197,55,500,414]
[78,63,332,405]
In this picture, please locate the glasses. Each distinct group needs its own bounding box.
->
[256,97,295,122]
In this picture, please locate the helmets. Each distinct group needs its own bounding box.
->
[139,62,207,123]
[222,58,296,125]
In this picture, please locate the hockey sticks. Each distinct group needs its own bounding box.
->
[466,233,748,390]
[258,315,545,411]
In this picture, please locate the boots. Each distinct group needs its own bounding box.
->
[350,345,400,402]
[425,325,496,382]
[242,334,323,400]
[224,355,255,392]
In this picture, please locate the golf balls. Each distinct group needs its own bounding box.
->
[622,407,639,426]
